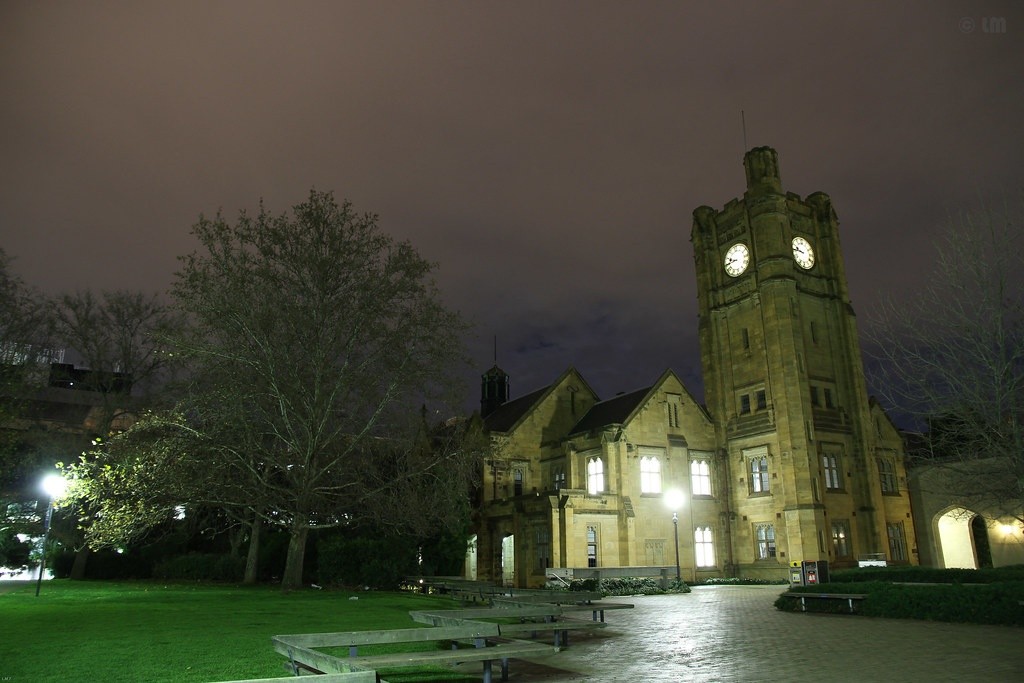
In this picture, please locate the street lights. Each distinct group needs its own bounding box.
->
[664,490,685,581]
[35,474,67,596]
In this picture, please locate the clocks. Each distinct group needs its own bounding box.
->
[792,236,817,272]
[721,240,752,279]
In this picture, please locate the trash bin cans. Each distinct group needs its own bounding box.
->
[803,561,829,585]
[788,560,806,588]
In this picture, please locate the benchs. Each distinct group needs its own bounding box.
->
[782,592,868,614]
[226,576,635,683]
